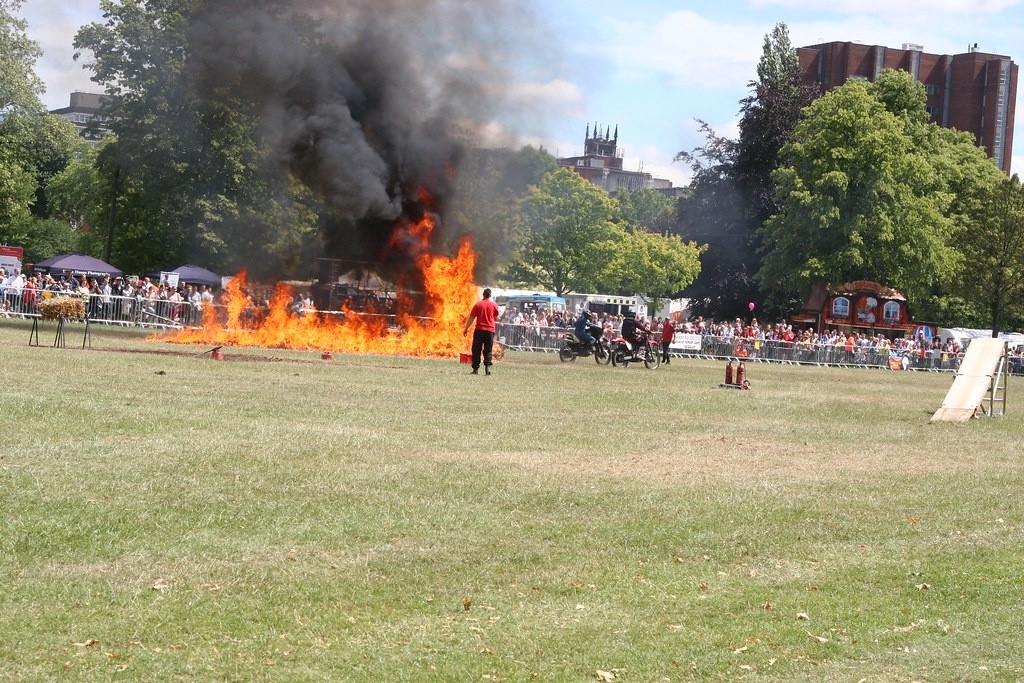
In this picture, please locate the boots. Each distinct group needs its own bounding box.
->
[583,341,592,354]
[636,346,646,361]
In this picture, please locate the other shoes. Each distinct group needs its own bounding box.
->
[470,370,478,375]
[665,362,670,365]
[661,360,665,363]
[485,371,491,375]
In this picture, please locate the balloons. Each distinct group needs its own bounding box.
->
[749,303,754,309]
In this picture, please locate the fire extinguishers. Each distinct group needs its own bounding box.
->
[736,357,747,384]
[725,356,734,384]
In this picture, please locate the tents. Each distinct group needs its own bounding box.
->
[146,256,226,289]
[32,253,123,280]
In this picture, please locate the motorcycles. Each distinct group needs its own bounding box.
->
[611,327,660,370]
[557,324,611,365]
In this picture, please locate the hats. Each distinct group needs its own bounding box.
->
[64,269,73,272]
[665,317,670,320]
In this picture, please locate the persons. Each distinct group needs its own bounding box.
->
[657,318,677,364]
[463,288,499,374]
[346,294,396,325]
[496,307,1024,377]
[621,309,652,361]
[0,268,319,332]
[574,309,596,355]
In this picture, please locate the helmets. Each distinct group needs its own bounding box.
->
[582,309,591,319]
[625,308,636,319]
